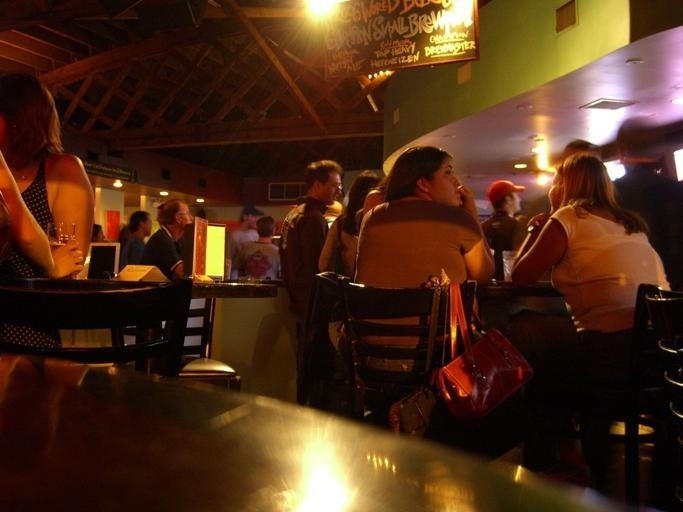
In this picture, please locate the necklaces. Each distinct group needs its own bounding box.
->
[6,163,30,181]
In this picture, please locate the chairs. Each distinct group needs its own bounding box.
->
[1,275,682,512]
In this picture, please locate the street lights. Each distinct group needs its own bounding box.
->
[432,277,535,431]
[388,283,478,453]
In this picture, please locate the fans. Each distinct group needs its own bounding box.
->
[324,0,480,81]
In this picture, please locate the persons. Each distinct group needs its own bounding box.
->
[353,146,495,372]
[0,149,83,281]
[89,159,385,315]
[479,141,671,494]
[608,116,683,296]
[0,73,95,357]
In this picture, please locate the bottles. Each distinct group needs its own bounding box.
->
[487,179,526,203]
[244,205,264,217]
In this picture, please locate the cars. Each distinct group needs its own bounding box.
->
[45,222,75,254]
[501,250,516,281]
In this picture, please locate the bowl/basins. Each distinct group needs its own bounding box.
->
[6,163,30,181]
